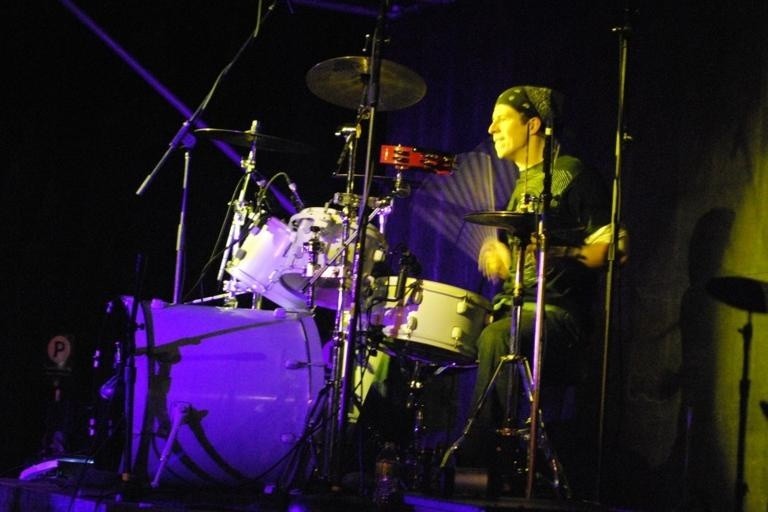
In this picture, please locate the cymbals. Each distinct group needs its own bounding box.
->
[462,210,586,233]
[194,128,307,153]
[306,56,427,112]
[707,274,768,315]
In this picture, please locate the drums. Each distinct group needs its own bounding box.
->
[380,143,460,177]
[84,296,328,490]
[371,275,495,366]
[224,206,389,314]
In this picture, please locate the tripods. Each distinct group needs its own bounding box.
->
[436,239,574,505]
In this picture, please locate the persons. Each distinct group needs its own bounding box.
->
[452,85,631,469]
